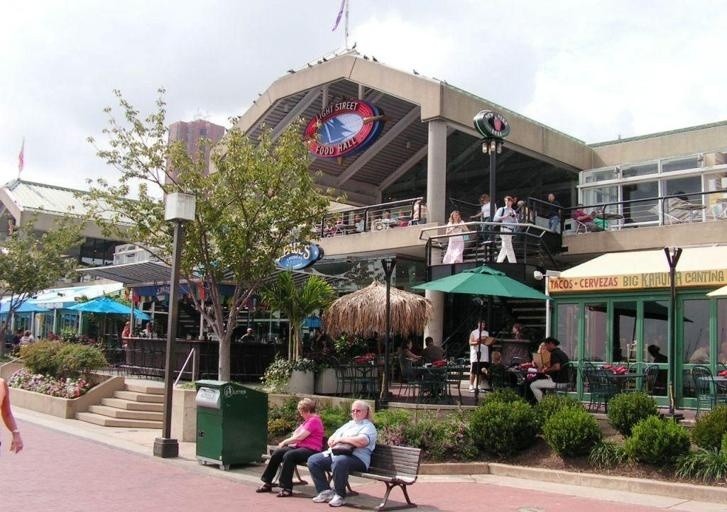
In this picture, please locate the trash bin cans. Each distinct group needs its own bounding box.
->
[195,379,267,470]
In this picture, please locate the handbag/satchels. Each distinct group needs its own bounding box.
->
[332,443,356,455]
[488,207,505,231]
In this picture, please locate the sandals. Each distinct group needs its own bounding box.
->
[276,488,292,497]
[256,483,272,493]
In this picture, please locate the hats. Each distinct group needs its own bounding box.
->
[545,337,560,345]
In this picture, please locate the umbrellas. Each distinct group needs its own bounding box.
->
[409,263,554,408]
[328,279,432,406]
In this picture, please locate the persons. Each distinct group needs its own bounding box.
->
[255,397,324,498]
[307,399,377,508]
[0,378,24,454]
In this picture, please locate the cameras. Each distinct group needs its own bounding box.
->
[510,213,515,218]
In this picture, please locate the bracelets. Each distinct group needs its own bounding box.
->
[11,429,20,434]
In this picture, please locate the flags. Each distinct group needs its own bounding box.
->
[330,0,346,32]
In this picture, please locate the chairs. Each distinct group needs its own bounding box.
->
[487,356,659,414]
[649,197,727,225]
[302,212,426,236]
[574,215,592,233]
[692,363,725,415]
[330,347,468,406]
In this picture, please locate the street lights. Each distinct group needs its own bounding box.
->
[663,244,683,419]
[378,257,397,408]
[151,189,198,459]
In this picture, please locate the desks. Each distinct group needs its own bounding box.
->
[594,213,623,231]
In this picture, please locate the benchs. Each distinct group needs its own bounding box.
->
[268,430,420,507]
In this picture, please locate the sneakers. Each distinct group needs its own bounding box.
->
[468,385,486,393]
[312,489,334,503]
[329,494,346,506]
